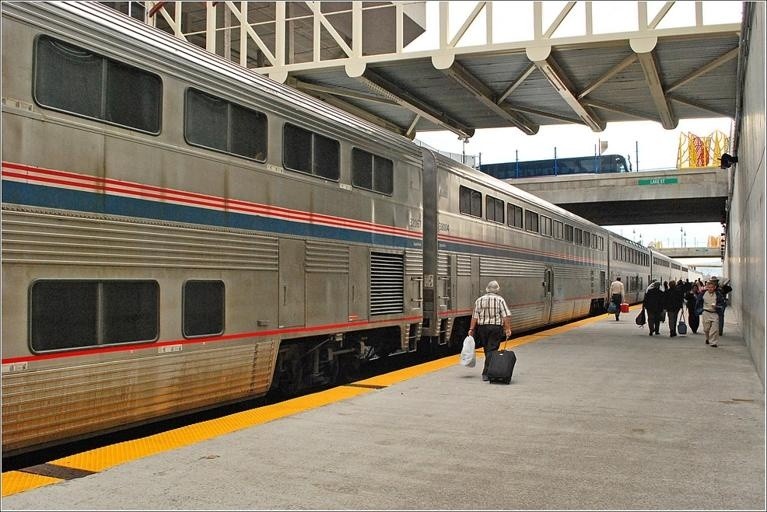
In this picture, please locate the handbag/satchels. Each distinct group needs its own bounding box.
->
[607,302,617,314]
[459,335,476,367]
[636,308,645,325]
[620,303,629,313]
[678,322,686,334]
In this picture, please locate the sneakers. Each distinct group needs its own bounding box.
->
[482,374,489,381]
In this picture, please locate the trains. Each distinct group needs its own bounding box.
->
[481,154,629,179]
[2,1,702,463]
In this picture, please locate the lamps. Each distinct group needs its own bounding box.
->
[721,154,738,170]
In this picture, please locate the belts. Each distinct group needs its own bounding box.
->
[705,310,717,313]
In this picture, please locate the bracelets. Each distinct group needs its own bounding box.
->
[469,327,474,331]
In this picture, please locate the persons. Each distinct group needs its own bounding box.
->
[646,276,726,347]
[609,277,624,320]
[468,280,513,382]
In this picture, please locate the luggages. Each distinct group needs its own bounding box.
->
[487,333,516,384]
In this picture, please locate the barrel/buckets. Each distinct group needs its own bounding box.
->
[620,300,629,313]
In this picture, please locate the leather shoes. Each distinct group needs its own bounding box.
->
[706,340,717,347]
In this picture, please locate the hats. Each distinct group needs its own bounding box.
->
[486,281,500,293]
[710,276,719,281]
